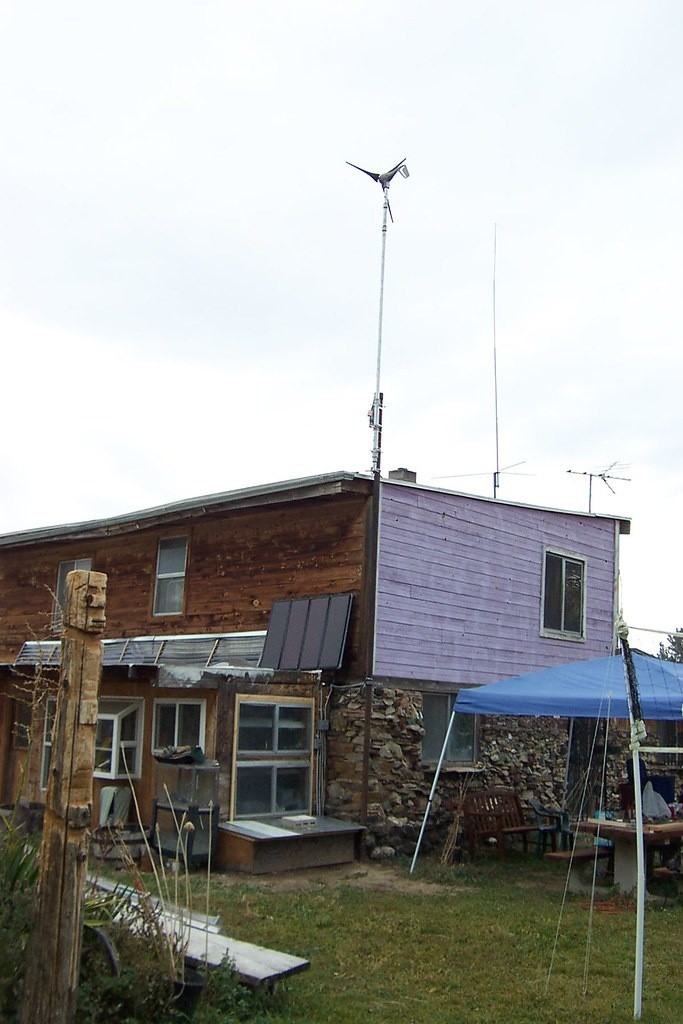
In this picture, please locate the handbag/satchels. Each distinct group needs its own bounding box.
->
[643,781,672,819]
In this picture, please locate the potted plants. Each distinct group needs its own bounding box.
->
[141,848,154,872]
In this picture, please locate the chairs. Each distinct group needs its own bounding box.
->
[529,799,576,858]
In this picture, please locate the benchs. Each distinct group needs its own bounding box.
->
[545,845,611,863]
[446,789,556,863]
[653,867,683,912]
[84,892,312,1000]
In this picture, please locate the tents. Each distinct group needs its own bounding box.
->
[409,651,683,874]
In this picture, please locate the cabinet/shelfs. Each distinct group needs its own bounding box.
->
[148,756,220,872]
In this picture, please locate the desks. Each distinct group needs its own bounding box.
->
[569,815,683,900]
